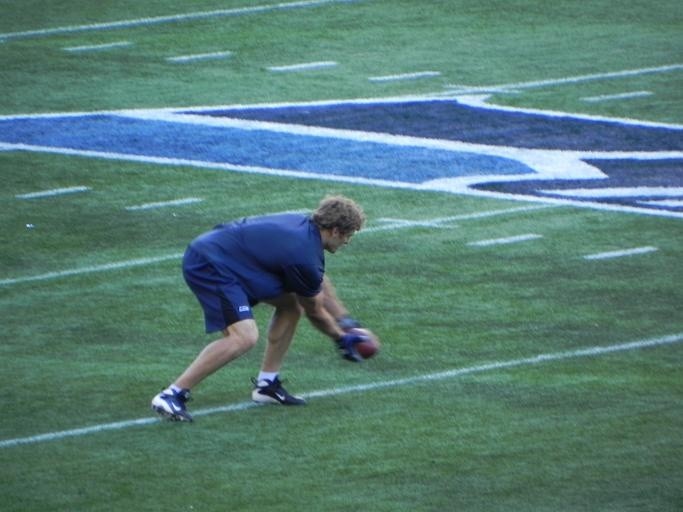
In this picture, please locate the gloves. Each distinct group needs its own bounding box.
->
[337,315,360,329]
[335,334,369,362]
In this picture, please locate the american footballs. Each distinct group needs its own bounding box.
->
[340,328,380,357]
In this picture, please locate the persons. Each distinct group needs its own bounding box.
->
[150,193,368,424]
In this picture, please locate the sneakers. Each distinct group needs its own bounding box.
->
[152,385,193,422]
[250,374,307,406]
